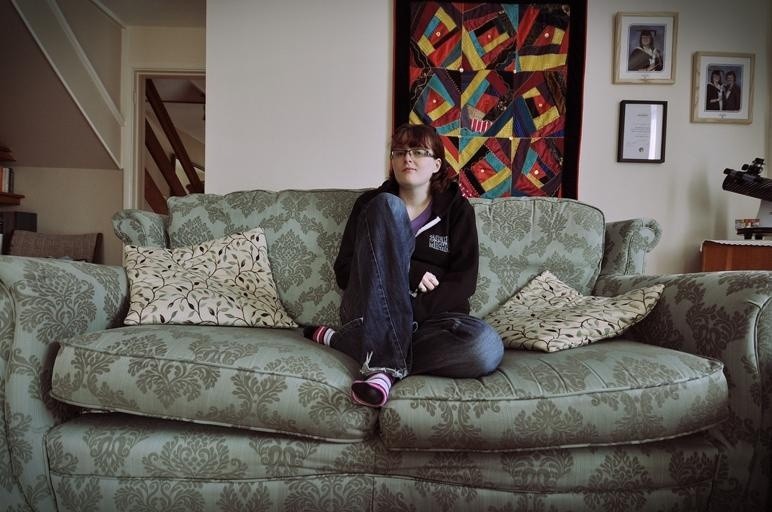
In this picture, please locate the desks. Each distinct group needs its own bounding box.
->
[702,240,771,272]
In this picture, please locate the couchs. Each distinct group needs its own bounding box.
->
[0,190,771,511]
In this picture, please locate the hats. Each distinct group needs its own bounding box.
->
[708,69,724,75]
[634,29,656,36]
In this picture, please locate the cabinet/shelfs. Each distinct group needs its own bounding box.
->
[0,211,38,255]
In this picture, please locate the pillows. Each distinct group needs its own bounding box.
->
[480,270,664,352]
[122,226,298,328]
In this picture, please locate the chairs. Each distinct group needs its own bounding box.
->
[7,229,101,263]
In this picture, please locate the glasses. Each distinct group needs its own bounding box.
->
[390,148,437,159]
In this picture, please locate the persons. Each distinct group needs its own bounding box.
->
[722,71,742,111]
[301,122,506,409]
[627,28,663,72]
[706,67,722,110]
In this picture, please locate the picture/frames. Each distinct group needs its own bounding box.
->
[615,99,669,164]
[388,1,586,200]
[691,51,755,123]
[612,10,678,85]
